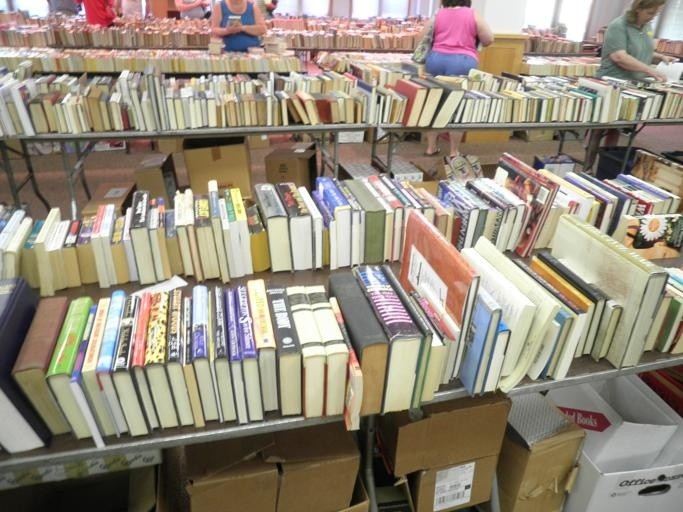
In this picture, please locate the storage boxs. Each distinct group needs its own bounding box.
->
[373,389,513,512]
[161,424,371,511]
[499,374,683,511]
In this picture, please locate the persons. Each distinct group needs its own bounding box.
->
[82,1,278,52]
[424,1,492,158]
[583,1,678,174]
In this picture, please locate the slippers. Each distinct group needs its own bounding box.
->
[425,147,466,160]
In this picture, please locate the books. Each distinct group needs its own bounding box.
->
[1,148,683,456]
[1,10,681,138]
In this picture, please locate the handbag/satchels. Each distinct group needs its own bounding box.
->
[411,25,434,64]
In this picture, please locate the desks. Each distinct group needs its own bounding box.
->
[0,45,683,475]
